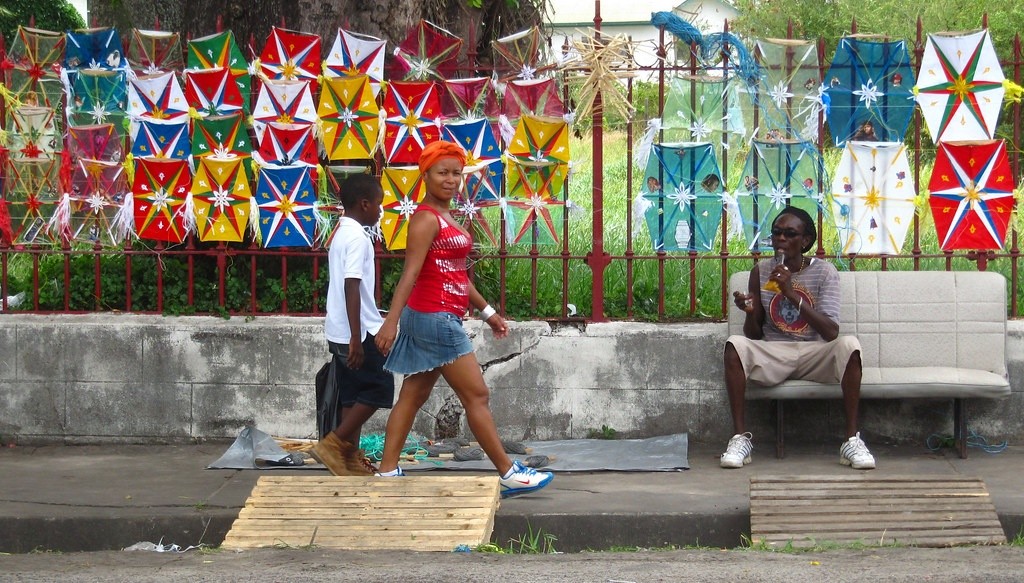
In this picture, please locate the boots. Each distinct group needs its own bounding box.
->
[347,447,379,476]
[311,430,353,477]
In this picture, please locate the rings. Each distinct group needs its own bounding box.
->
[776,273,780,277]
[783,265,788,271]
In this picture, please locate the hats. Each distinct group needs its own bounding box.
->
[419,140,468,175]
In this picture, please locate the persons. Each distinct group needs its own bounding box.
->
[375,141,553,500]
[720,208,876,470]
[309,173,394,478]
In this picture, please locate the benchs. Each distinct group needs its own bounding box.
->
[727,271,1012,457]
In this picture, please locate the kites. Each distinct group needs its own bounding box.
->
[0,13,1015,254]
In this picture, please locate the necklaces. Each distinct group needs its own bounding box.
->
[789,253,804,280]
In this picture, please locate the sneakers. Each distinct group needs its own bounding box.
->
[720,432,754,467]
[372,465,408,477]
[499,459,554,494]
[839,431,875,469]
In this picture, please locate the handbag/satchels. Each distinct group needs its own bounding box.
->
[316,351,343,440]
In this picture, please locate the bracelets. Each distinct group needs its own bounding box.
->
[798,297,803,311]
[479,304,496,321]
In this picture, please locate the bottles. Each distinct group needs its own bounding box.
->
[762,248,785,291]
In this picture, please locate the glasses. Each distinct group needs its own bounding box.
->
[771,227,805,238]
